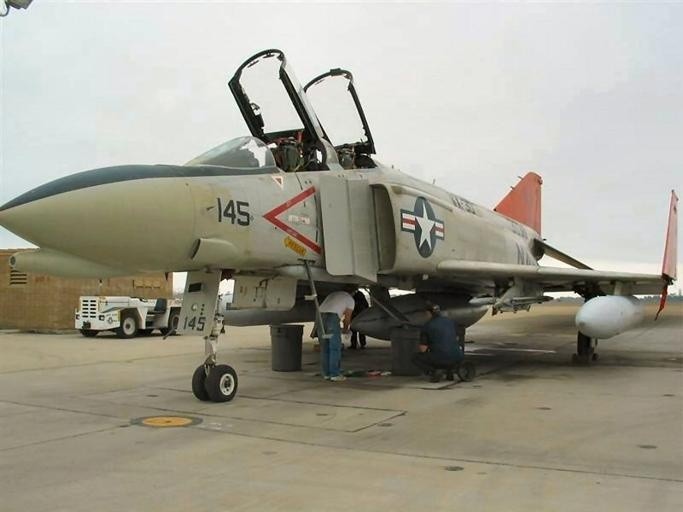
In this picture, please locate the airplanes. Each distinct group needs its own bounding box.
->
[0,47,681,405]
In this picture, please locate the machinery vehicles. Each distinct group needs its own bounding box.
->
[72,296,182,336]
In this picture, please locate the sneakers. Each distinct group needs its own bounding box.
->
[446,373,454,381]
[431,374,439,382]
[325,375,347,381]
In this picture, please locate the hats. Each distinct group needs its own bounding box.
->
[425,304,440,312]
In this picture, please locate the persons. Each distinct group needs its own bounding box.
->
[412,304,463,382]
[348,285,369,350]
[317,285,355,382]
